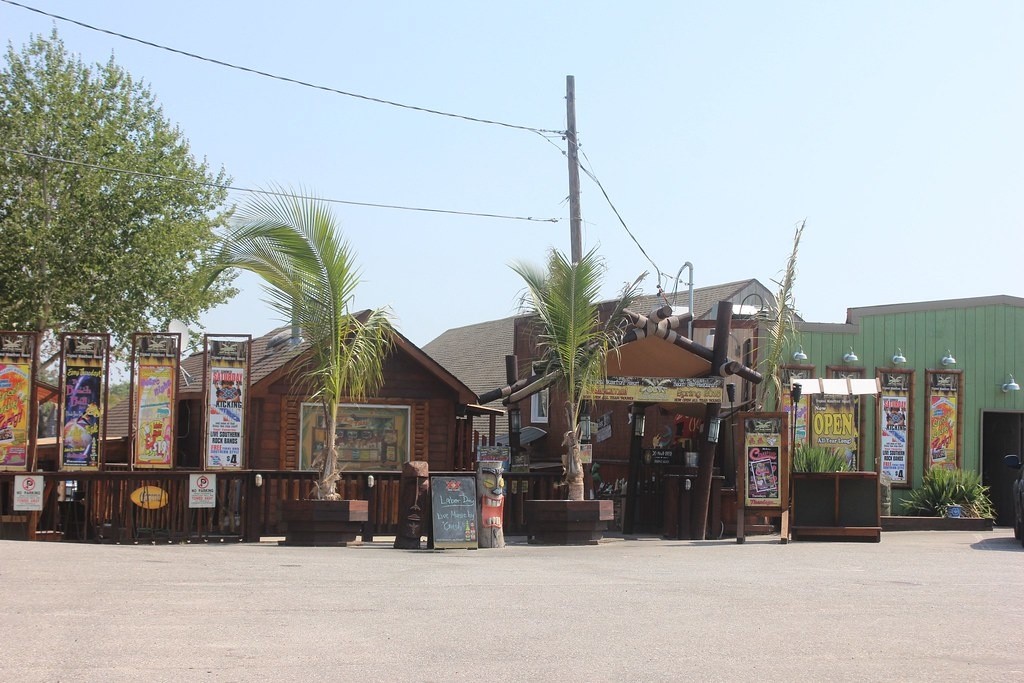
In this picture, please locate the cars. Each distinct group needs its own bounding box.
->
[1002,451,1024,555]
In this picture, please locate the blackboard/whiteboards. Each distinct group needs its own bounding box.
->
[430,472,479,549]
[745,433,781,506]
